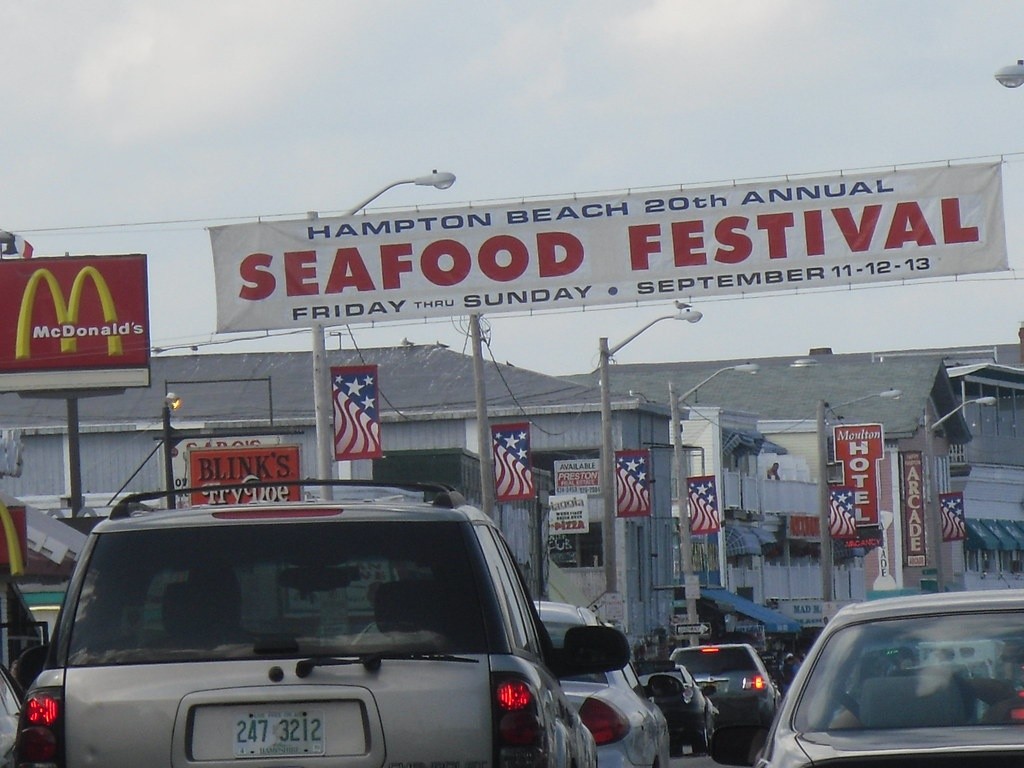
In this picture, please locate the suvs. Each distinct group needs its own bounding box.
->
[0,479,631,768]
[672,643,780,725]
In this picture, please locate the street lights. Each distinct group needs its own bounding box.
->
[925,397,995,593]
[815,390,903,597]
[598,306,703,597]
[668,365,762,626]
[308,172,457,500]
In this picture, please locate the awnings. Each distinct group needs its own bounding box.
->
[835,538,865,560]
[965,518,1024,550]
[700,589,801,633]
[725,525,778,556]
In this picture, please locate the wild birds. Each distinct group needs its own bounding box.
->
[673,300,692,312]
[400,337,415,346]
[151,346,166,355]
[437,341,449,348]
[506,361,515,367]
[192,346,198,352]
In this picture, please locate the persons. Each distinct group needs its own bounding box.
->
[767,463,780,480]
[779,653,798,696]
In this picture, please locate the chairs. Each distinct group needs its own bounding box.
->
[161,585,256,649]
[350,581,457,641]
[857,673,969,726]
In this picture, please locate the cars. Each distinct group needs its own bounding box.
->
[753,589,1023,768]
[533,601,671,768]
[632,660,719,758]
